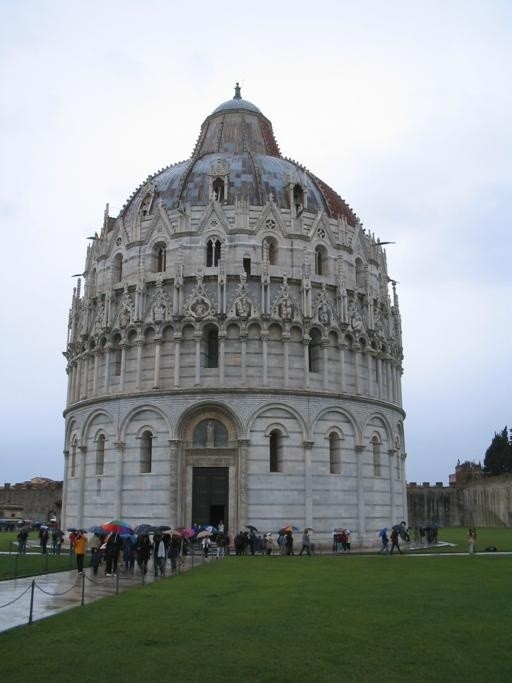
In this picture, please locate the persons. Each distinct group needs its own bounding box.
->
[299,532,312,555]
[17,520,64,555]
[69,531,88,576]
[91,288,398,348]
[332,532,351,552]
[91,530,189,576]
[413,525,438,544]
[192,521,294,559]
[390,530,403,554]
[466,527,476,554]
[472,528,477,552]
[378,532,389,552]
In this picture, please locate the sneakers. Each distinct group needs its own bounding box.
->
[78,570,180,577]
